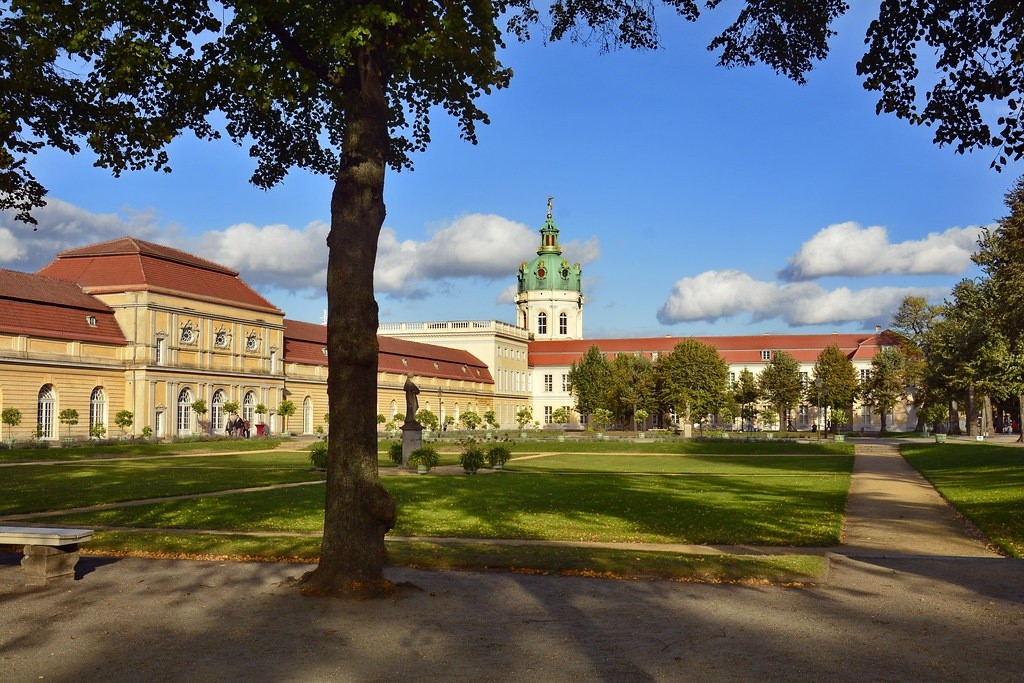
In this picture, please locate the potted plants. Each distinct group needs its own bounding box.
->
[826,408,849,442]
[191,397,211,439]
[740,408,760,441]
[114,409,134,443]
[89,420,107,444]
[923,402,950,444]
[253,403,268,438]
[516,406,534,439]
[59,408,80,444]
[221,399,243,438]
[718,407,732,439]
[757,408,780,440]
[587,407,615,442]
[1,406,23,449]
[633,408,649,438]
[305,408,512,475]
[276,399,298,439]
[915,405,932,438]
[141,424,153,442]
[550,407,572,442]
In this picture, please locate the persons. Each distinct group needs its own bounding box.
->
[993,414,1018,435]
[226,415,250,439]
[811,419,831,432]
[404,374,421,423]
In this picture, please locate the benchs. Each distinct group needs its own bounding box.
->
[0,525,96,587]
